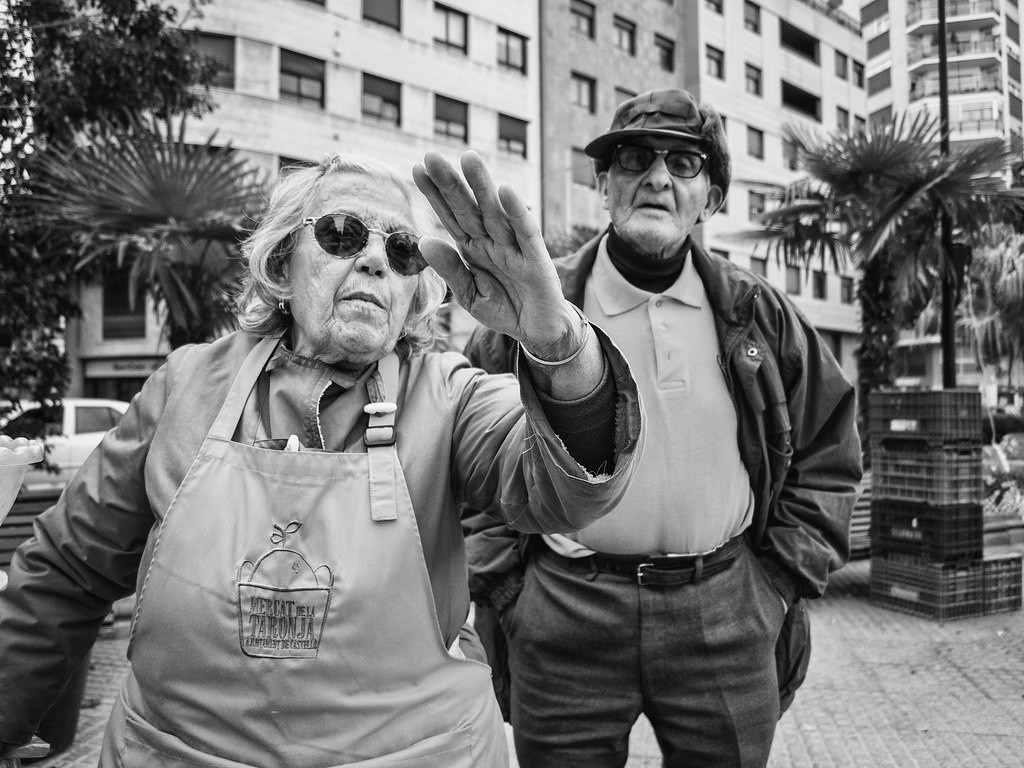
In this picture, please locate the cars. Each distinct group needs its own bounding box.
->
[1,398,131,501]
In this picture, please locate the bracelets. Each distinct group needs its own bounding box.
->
[519,298,589,366]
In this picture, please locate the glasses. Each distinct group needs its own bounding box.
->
[606,143,712,187]
[298,213,428,276]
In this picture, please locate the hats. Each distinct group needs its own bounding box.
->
[583,89,732,226]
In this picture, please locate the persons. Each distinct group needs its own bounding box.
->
[461,89,863,768]
[0,151,648,768]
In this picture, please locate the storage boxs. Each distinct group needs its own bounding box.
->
[867,389,1022,624]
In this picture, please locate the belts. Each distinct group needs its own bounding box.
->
[541,531,745,588]
